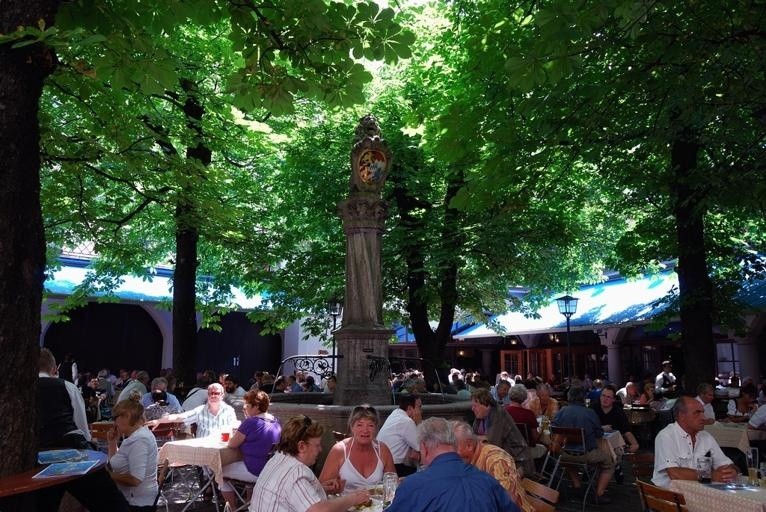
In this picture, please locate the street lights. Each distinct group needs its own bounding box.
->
[554,291,579,386]
[327,295,343,380]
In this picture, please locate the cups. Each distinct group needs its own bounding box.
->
[696,457,714,484]
[374,472,399,509]
[745,446,761,489]
[221,426,230,442]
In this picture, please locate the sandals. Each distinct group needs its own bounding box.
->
[614,465,624,484]
[204,492,211,502]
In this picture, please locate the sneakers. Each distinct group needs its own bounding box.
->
[596,492,612,504]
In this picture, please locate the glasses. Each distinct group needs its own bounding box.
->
[112,416,118,421]
[297,415,311,443]
[353,407,376,415]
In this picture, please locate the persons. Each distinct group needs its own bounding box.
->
[32,345,766,511]
